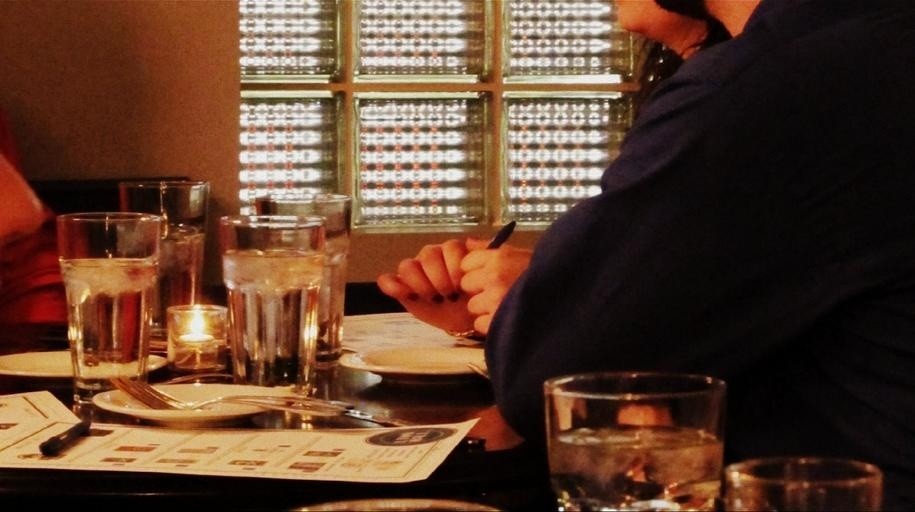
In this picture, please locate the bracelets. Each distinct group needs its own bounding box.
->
[443,328,478,337]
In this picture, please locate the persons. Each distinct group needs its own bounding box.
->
[483,1,915,510]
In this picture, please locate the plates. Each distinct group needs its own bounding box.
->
[90,381,294,427]
[336,342,492,383]
[0,351,168,377]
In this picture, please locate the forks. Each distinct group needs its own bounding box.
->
[111,373,356,419]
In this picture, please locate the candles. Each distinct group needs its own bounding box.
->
[174,304,218,370]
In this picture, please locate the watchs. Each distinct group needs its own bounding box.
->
[375,3,736,352]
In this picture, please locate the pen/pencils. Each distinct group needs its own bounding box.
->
[39,422,90,455]
[488,220,518,252]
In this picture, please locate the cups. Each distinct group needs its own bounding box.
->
[722,454,884,511]
[542,369,723,510]
[214,212,328,397]
[252,195,356,377]
[113,178,211,357]
[163,303,228,375]
[52,210,163,406]
